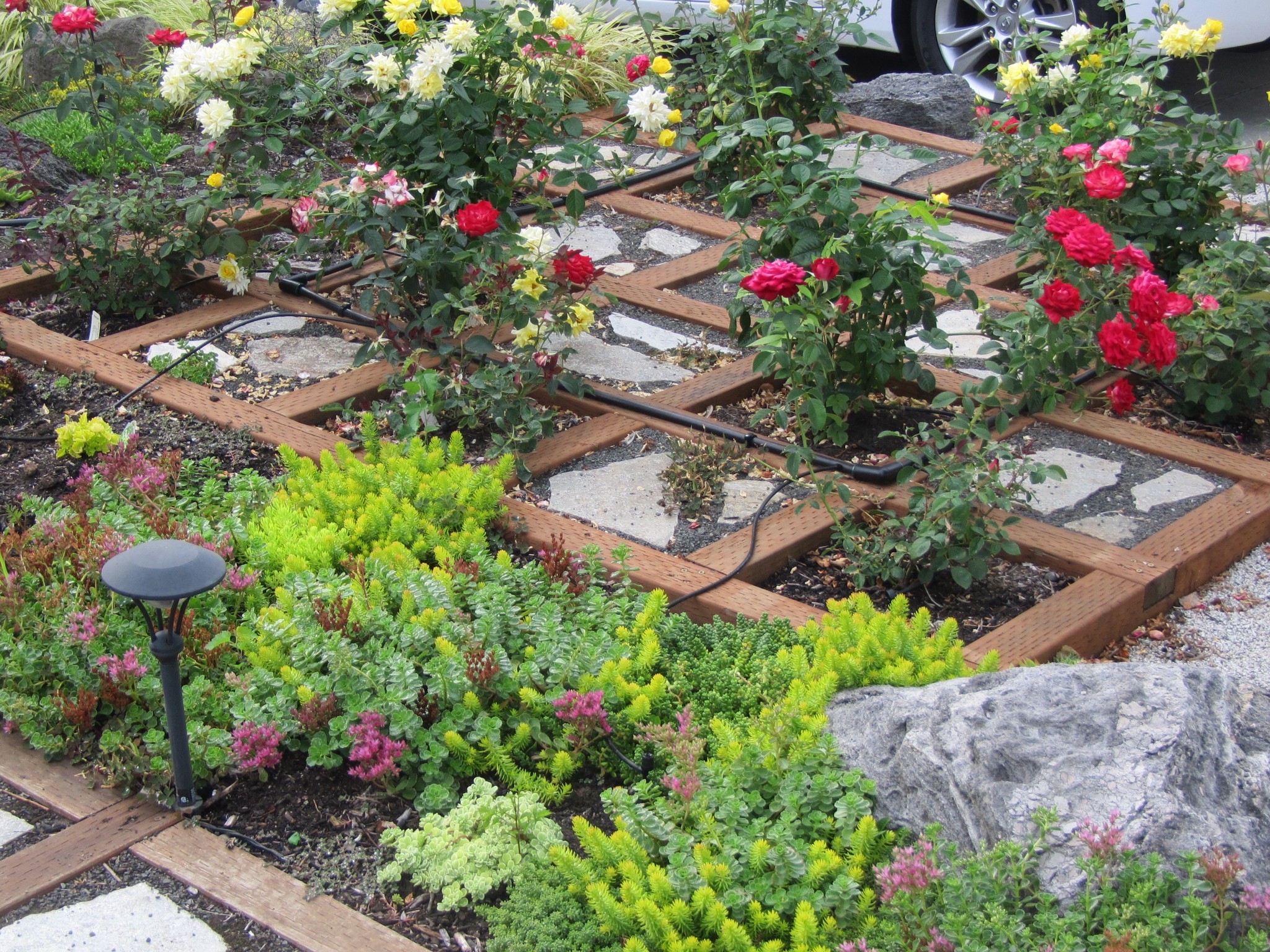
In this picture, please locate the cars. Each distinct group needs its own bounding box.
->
[466,0,1270,122]
